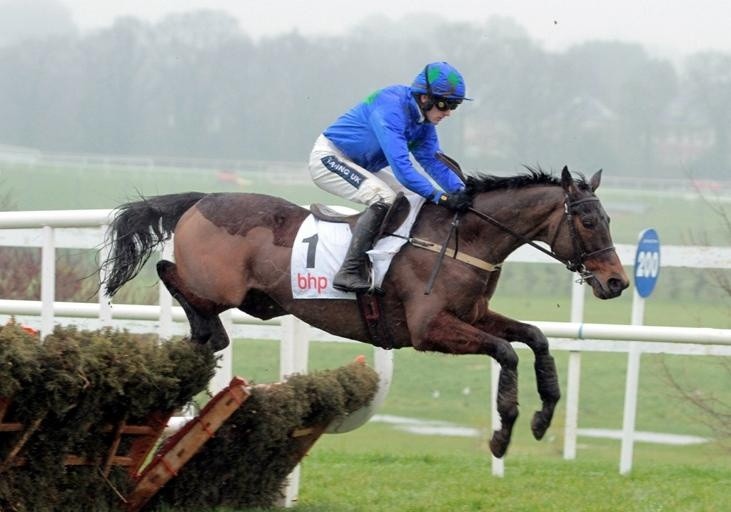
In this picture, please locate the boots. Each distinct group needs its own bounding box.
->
[334,201,391,290]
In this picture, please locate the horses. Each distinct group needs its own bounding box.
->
[77,162,630,459]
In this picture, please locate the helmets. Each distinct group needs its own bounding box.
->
[411,62,466,104]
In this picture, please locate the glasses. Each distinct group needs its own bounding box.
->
[425,65,458,112]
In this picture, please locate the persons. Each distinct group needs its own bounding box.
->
[309,61,473,292]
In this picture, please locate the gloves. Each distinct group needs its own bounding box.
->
[439,190,474,214]
